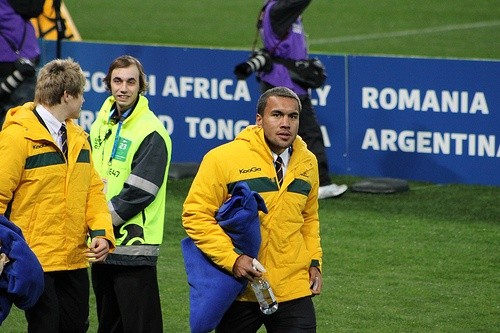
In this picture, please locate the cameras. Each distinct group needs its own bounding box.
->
[233,50,273,79]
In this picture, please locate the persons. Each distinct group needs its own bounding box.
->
[0,0,39,117]
[89,55,172,333]
[182,87,322,333]
[0,59,115,333]
[256,0,347,200]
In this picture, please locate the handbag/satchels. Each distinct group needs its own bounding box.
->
[288,58,327,90]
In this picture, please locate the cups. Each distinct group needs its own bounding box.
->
[251,267,278,314]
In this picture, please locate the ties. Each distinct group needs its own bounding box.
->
[60,125,68,159]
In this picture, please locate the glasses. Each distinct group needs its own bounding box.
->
[276,157,285,186]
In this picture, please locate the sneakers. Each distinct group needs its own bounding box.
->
[318,183,348,199]
